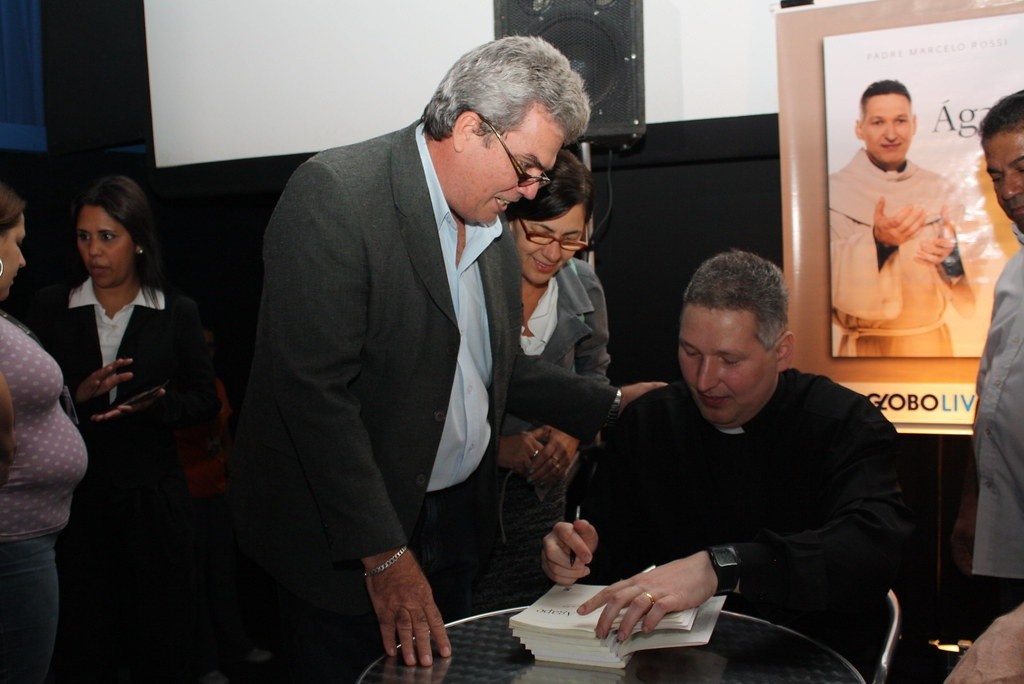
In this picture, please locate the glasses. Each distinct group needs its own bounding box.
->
[467,108,551,189]
[517,214,590,251]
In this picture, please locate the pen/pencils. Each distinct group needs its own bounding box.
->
[569,505,581,565]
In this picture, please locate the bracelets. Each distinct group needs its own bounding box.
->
[601,388,622,429]
[355,546,407,578]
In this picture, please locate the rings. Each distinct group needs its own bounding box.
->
[642,591,655,606]
[533,449,539,456]
[552,455,559,461]
[555,465,561,470]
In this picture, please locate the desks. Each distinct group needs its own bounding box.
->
[356,604,868,684]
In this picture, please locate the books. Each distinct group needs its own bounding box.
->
[508,564,725,667]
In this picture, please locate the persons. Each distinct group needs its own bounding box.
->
[173,328,272,678]
[33,173,220,684]
[0,179,88,684]
[539,257,922,640]
[476,144,612,611]
[942,89,1023,684]
[224,34,669,684]
[827,80,980,358]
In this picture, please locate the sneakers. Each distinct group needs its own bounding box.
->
[239,647,272,662]
[198,669,229,684]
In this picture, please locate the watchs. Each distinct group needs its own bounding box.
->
[701,543,740,596]
